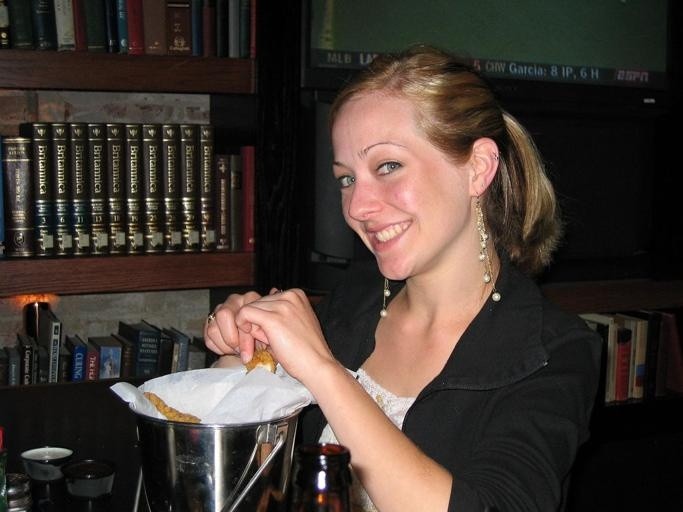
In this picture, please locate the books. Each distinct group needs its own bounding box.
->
[578,309,681,406]
[18,123,258,255]
[1,302,220,386]
[2,1,257,61]
[2,137,36,258]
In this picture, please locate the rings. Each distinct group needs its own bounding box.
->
[207,312,216,324]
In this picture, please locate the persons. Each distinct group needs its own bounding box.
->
[201,39,604,512]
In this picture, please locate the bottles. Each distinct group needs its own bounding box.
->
[295,443,356,511]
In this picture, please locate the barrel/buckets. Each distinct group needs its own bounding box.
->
[131,406,306,512]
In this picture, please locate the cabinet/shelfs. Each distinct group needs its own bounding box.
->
[0,1,317,470]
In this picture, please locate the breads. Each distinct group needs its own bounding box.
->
[246,351,276,372]
[143,391,200,424]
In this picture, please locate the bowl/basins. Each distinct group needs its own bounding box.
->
[64,457,115,498]
[21,447,75,482]
